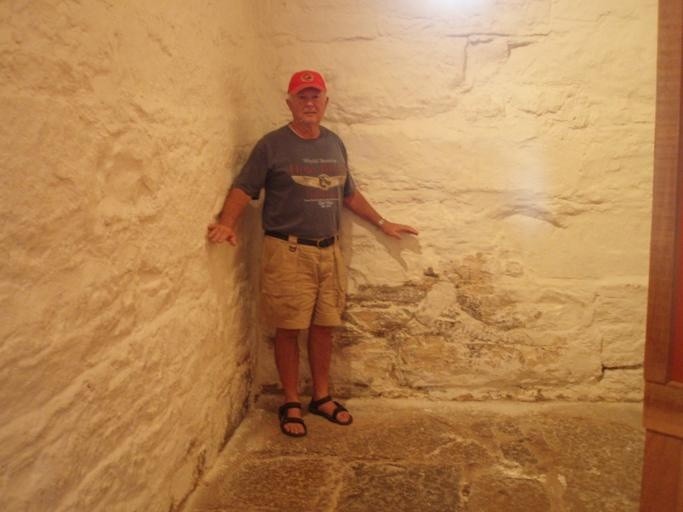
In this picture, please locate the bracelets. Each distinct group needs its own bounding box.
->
[375,217,385,229]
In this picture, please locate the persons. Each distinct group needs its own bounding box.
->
[205,69,419,438]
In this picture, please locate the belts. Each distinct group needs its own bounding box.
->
[265,229,339,249]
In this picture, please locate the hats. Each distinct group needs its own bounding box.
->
[288,70,327,96]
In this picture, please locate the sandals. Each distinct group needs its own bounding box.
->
[278,402,307,436]
[308,395,352,425]
